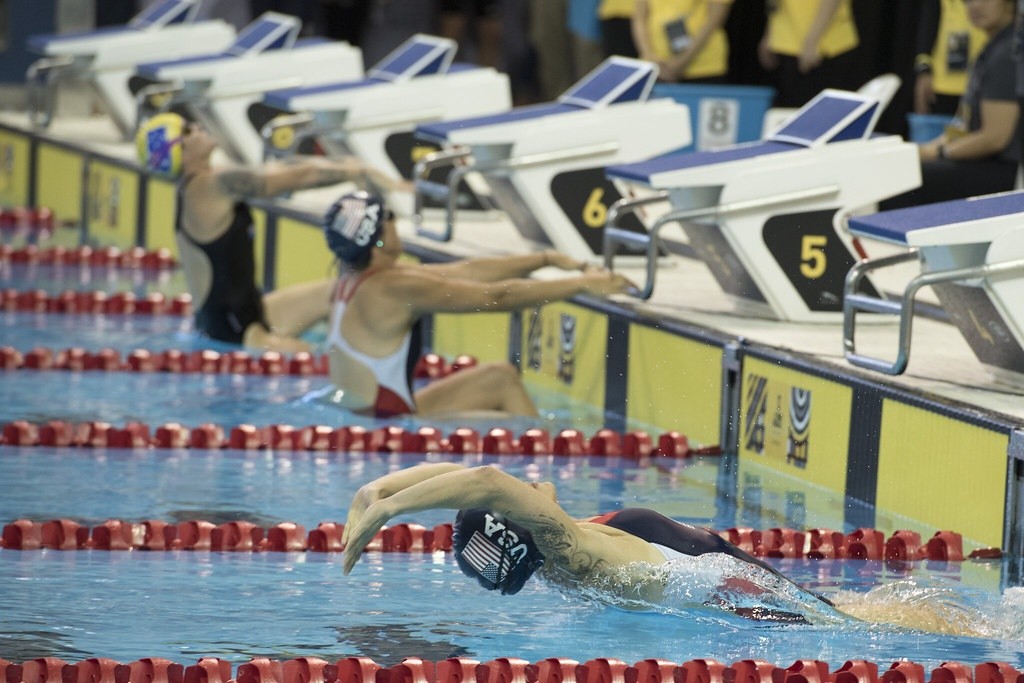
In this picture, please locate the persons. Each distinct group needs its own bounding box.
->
[342,463,835,608]
[878,0,1024,210]
[326,190,641,417]
[632,0,730,86]
[136,114,392,352]
[758,0,860,108]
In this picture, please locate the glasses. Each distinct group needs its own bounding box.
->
[336,210,394,252]
[151,117,195,171]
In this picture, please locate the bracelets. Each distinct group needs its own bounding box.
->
[939,143,945,158]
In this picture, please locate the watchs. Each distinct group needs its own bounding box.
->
[916,63,932,74]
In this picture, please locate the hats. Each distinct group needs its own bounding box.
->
[451,507,544,595]
[134,112,182,178]
[324,189,383,268]
